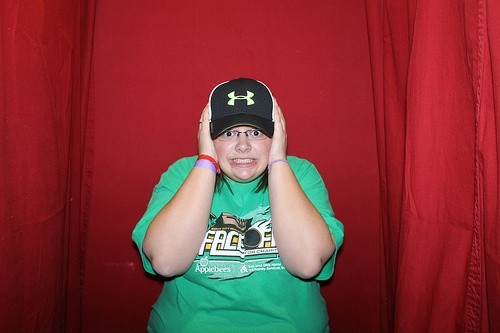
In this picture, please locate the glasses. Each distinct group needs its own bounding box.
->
[215,128,268,142]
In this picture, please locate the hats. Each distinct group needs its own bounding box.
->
[208,77,276,138]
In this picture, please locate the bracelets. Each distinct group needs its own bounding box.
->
[196,155,221,175]
[194,159,217,173]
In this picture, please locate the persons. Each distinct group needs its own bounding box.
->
[133,77,345,333]
